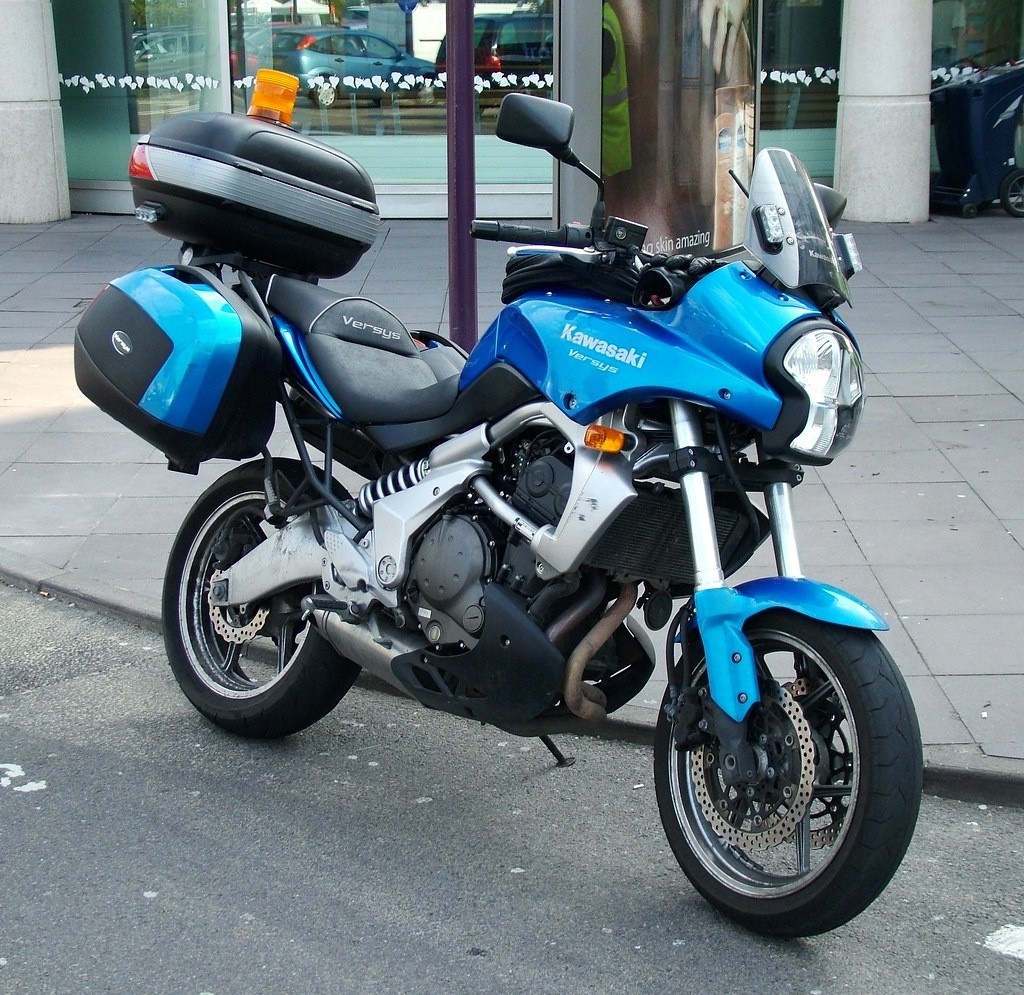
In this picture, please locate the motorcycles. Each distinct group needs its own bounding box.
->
[61,98,939,943]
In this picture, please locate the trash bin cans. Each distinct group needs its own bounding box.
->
[930,58,1024,219]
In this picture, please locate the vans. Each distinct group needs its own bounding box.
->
[339,5,372,30]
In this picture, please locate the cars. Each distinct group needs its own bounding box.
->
[255,28,444,110]
[129,23,208,86]
[229,21,291,78]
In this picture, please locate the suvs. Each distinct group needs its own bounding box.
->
[433,14,555,116]
